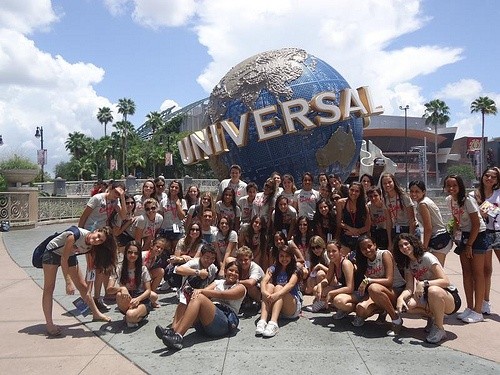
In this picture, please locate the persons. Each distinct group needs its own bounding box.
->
[108,242,151,328]
[396,233,461,342]
[355,233,407,337]
[155,261,247,350]
[313,240,369,320]
[78,164,500,311]
[42,224,118,335]
[255,244,303,337]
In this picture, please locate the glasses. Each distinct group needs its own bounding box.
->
[128,251,139,256]
[126,202,134,205]
[145,207,155,211]
[191,228,199,232]
[310,245,320,251]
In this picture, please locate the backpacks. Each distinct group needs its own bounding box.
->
[32,226,80,268]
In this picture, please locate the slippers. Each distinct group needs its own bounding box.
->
[49,326,65,336]
[92,316,110,322]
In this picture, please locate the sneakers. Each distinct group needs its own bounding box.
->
[482,301,491,315]
[263,322,279,337]
[425,321,431,332]
[155,325,174,339]
[255,320,267,334]
[457,308,472,318]
[462,310,483,323]
[332,311,349,320]
[94,296,111,311]
[162,333,183,349]
[352,315,367,326]
[426,327,446,343]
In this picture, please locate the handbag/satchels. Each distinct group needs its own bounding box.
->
[428,232,451,250]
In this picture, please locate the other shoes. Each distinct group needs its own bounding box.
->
[386,319,403,336]
[160,282,171,291]
[376,312,387,324]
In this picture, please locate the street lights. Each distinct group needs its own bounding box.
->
[399,105,409,191]
[35,126,43,189]
